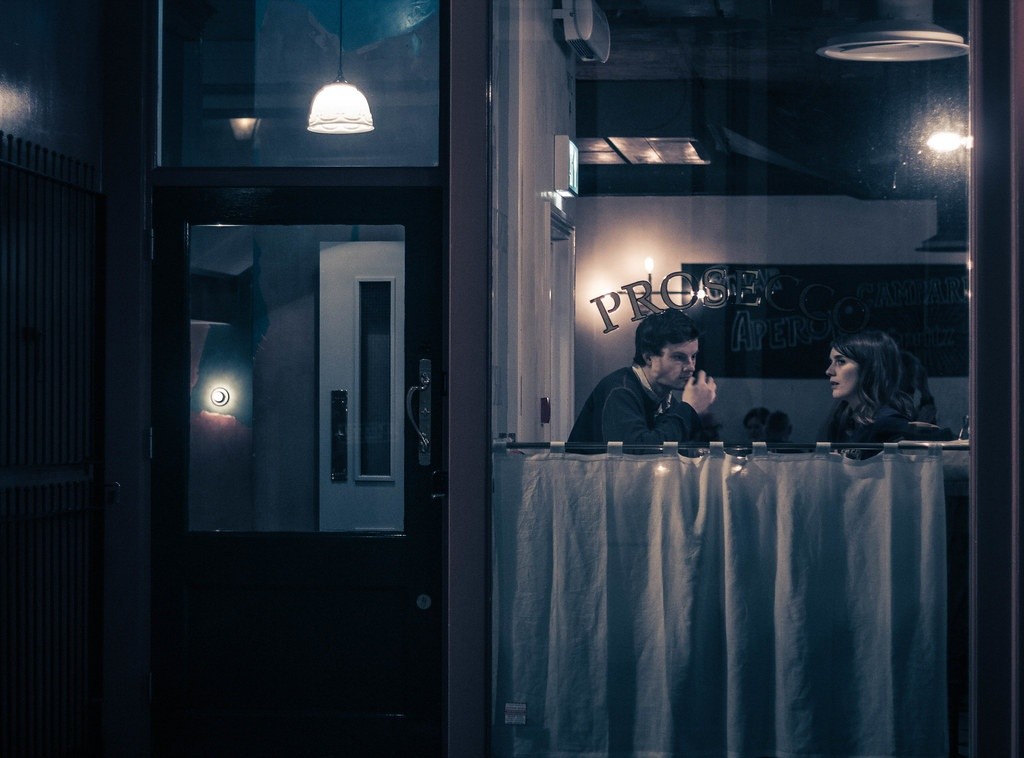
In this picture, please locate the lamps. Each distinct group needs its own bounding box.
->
[307,0,376,134]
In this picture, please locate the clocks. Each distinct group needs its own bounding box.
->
[555,134,580,200]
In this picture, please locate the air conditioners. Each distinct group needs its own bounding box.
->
[552,0,611,64]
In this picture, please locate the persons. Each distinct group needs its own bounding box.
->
[565,307,717,455]
[697,406,804,454]
[818,328,942,460]
